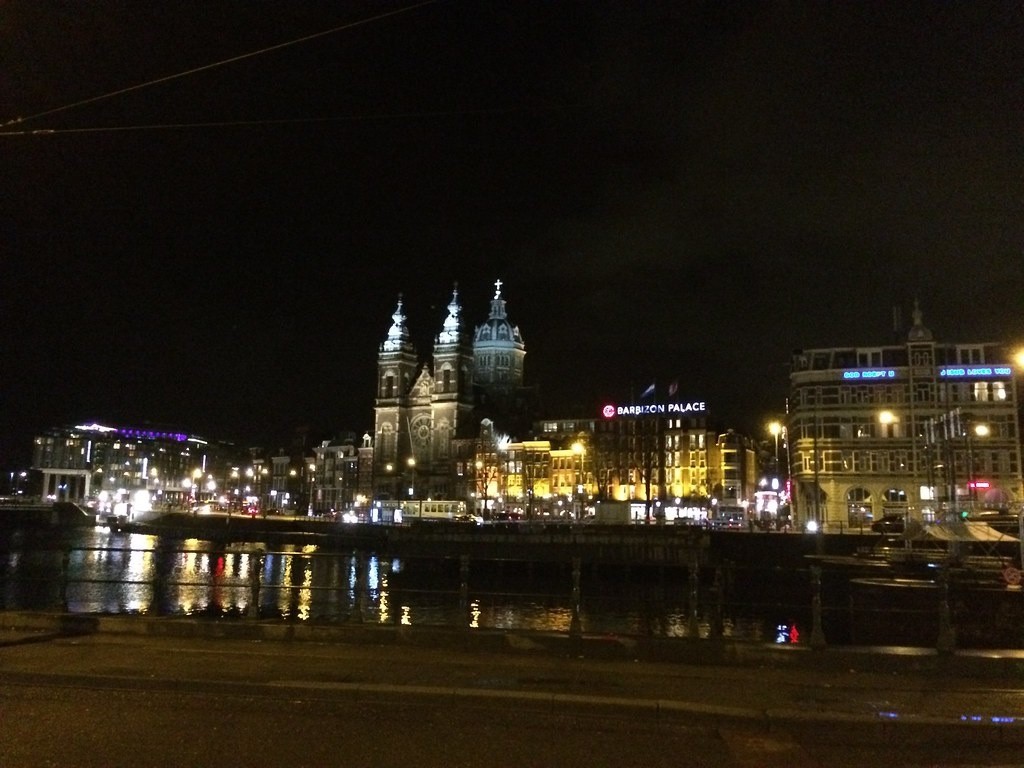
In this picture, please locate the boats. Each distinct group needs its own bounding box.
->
[802,524,1024,609]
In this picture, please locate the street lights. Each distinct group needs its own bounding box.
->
[386,463,401,510]
[769,418,783,531]
[307,463,316,518]
[12,471,27,507]
[476,461,490,520]
[570,441,586,520]
[408,457,415,500]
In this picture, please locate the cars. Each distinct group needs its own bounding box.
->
[493,511,527,519]
[870,514,924,533]
[716,518,741,531]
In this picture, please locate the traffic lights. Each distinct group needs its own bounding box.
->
[969,482,990,488]
